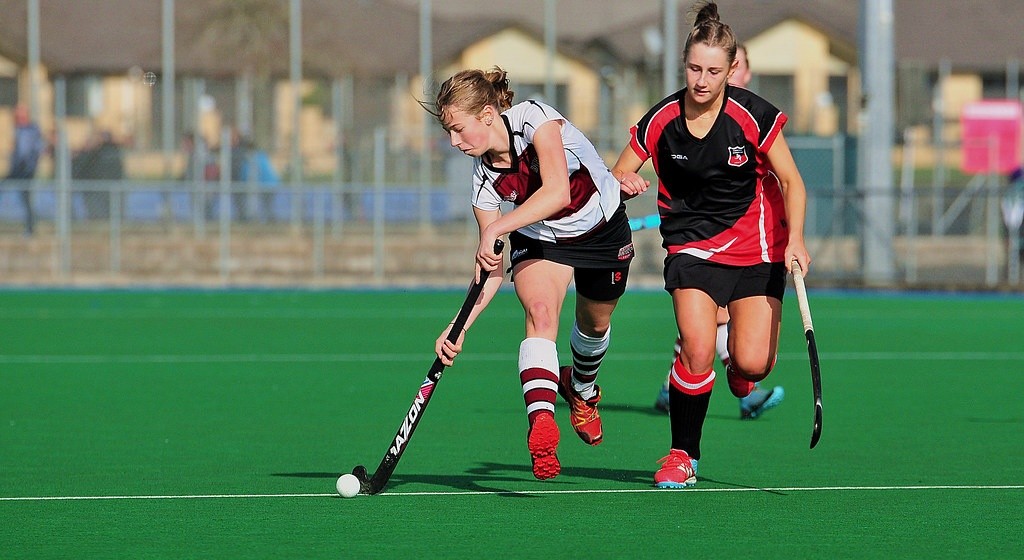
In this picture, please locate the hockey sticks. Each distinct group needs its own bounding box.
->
[791,259,822,448]
[351,239,505,495]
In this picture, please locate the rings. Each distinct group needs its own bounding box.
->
[621,177,627,183]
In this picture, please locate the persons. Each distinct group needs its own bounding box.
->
[44,128,126,219]
[434,69,636,482]
[653,43,785,422]
[177,124,282,220]
[4,104,46,235]
[607,2,812,489]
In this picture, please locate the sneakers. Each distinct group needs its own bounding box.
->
[654,449,698,488]
[558,366,604,447]
[654,386,670,416]
[527,413,561,480]
[740,383,785,421]
[726,365,755,398]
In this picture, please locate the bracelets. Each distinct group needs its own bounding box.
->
[449,323,466,332]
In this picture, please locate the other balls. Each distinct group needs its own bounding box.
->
[337,475,359,495]
